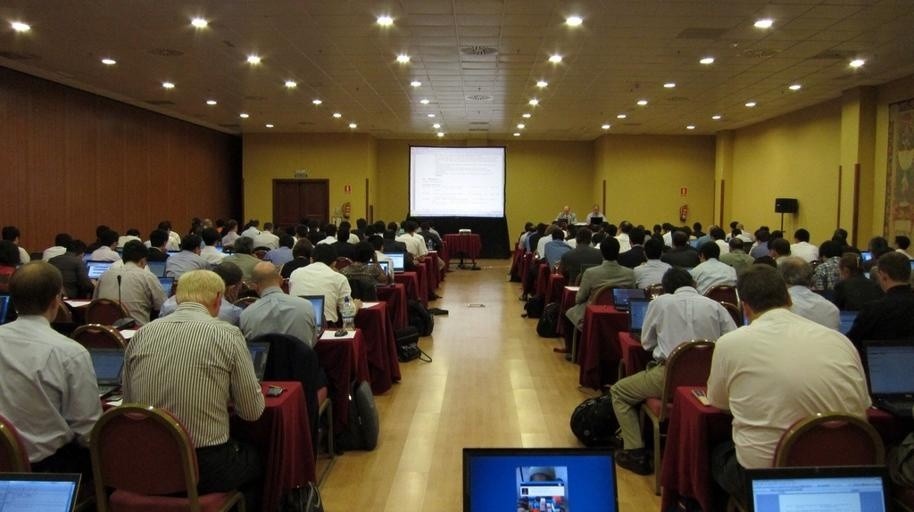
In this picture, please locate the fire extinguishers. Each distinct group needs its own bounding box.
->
[343,202,351,218]
[680,205,688,221]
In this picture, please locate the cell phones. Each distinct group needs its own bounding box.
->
[335,329,347,337]
[267,388,282,396]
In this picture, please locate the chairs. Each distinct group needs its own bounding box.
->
[0,415,32,474]
[57,301,73,320]
[88,401,246,512]
[69,324,126,350]
[774,410,887,470]
[84,298,131,327]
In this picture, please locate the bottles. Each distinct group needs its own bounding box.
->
[427,238,432,251]
[340,297,354,330]
[388,267,394,286]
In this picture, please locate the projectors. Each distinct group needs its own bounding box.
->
[458,229,472,235]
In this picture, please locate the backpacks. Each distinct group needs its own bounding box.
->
[571,395,624,448]
[394,299,434,362]
[521,294,559,337]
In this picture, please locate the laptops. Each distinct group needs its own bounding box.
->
[860,339,914,416]
[839,310,860,334]
[87,348,125,399]
[298,295,324,338]
[861,251,914,280]
[0,293,14,326]
[591,217,603,225]
[0,472,82,512]
[367,252,405,287]
[741,464,893,512]
[246,341,270,387]
[82,246,225,298]
[463,448,619,512]
[628,298,654,342]
[611,288,646,312]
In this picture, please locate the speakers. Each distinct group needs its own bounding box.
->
[774,197,798,213]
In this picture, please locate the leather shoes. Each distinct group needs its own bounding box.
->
[616,452,650,474]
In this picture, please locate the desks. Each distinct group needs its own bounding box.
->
[444,233,480,269]
[513,242,896,390]
[660,384,894,512]
[229,241,446,448]
[231,379,314,512]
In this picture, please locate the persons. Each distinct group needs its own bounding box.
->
[584,205,607,225]
[2,217,443,350]
[556,206,577,225]
[2,259,116,500]
[519,221,911,475]
[710,264,872,509]
[121,268,266,507]
